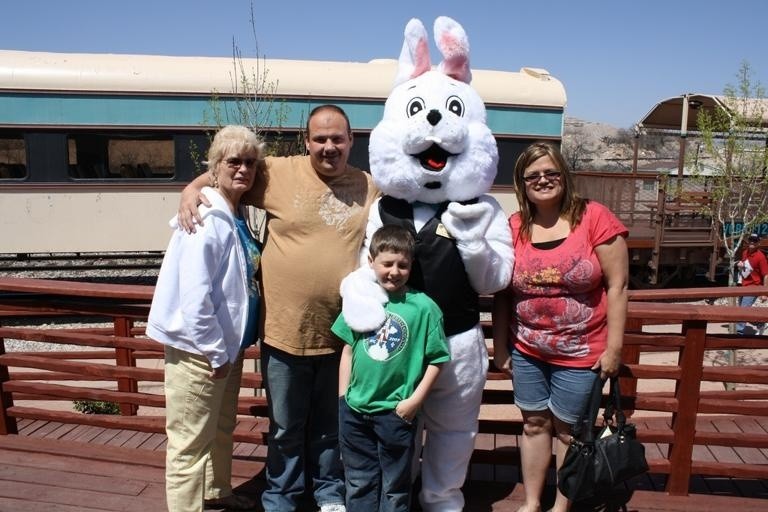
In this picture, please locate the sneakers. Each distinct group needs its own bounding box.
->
[204,492,257,510]
[321,504,346,512]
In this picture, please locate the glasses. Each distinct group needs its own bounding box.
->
[218,156,257,167]
[522,170,563,183]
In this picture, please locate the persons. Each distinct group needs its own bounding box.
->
[490,141,633,512]
[736,232,768,336]
[143,121,263,512]
[175,104,383,512]
[329,223,454,512]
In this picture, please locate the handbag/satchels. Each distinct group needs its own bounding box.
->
[557,422,649,505]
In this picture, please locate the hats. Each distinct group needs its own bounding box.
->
[748,234,761,241]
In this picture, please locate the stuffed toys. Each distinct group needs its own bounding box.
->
[356,15,516,511]
